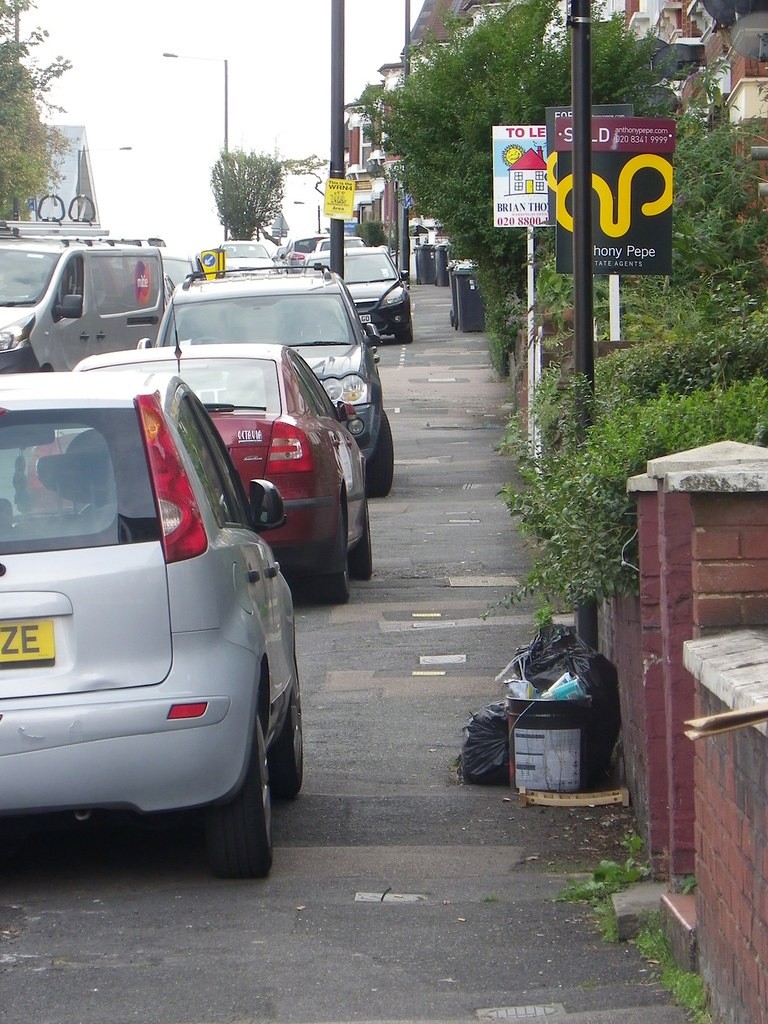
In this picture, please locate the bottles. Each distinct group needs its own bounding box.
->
[541,679,585,699]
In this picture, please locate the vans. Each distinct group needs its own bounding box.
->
[0,216,166,374]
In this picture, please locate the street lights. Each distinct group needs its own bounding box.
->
[294,200,321,233]
[163,53,230,240]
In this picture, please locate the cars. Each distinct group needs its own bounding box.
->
[275,236,365,277]
[158,247,206,287]
[302,246,414,343]
[219,241,279,279]
[27,344,374,605]
[0,370,310,882]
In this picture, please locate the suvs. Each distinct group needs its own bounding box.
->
[141,262,397,497]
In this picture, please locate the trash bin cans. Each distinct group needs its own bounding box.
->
[413,243,458,287]
[445,264,486,333]
[506,693,591,793]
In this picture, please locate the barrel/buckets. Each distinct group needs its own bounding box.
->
[506,696,592,795]
